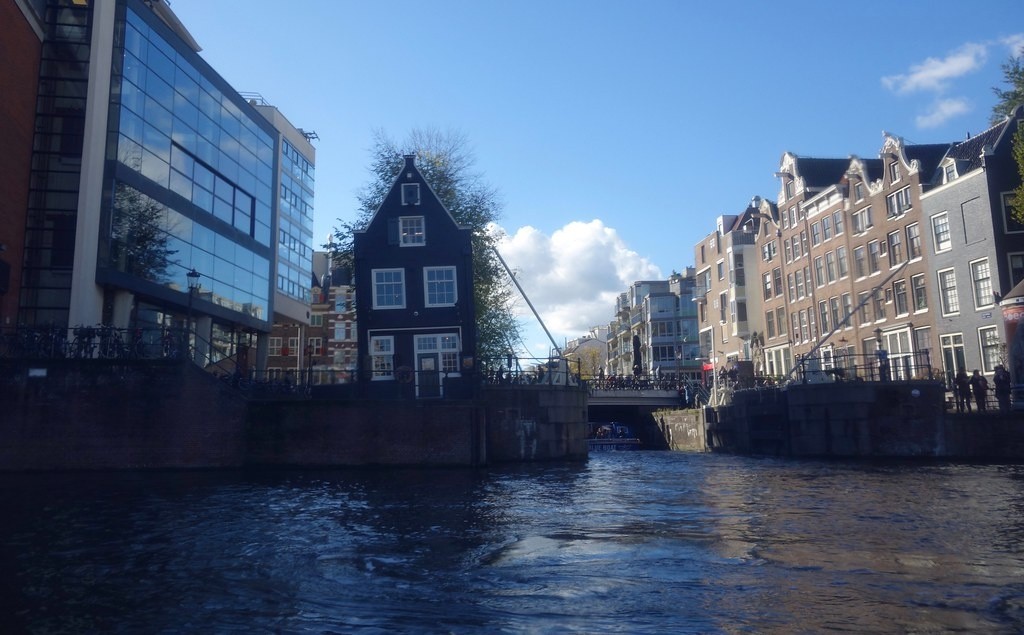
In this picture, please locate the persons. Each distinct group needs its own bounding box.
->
[969,370,989,411]
[679,386,686,410]
[992,364,1011,411]
[654,364,662,390]
[954,367,972,412]
[488,365,544,384]
[598,367,604,390]
[632,365,640,390]
